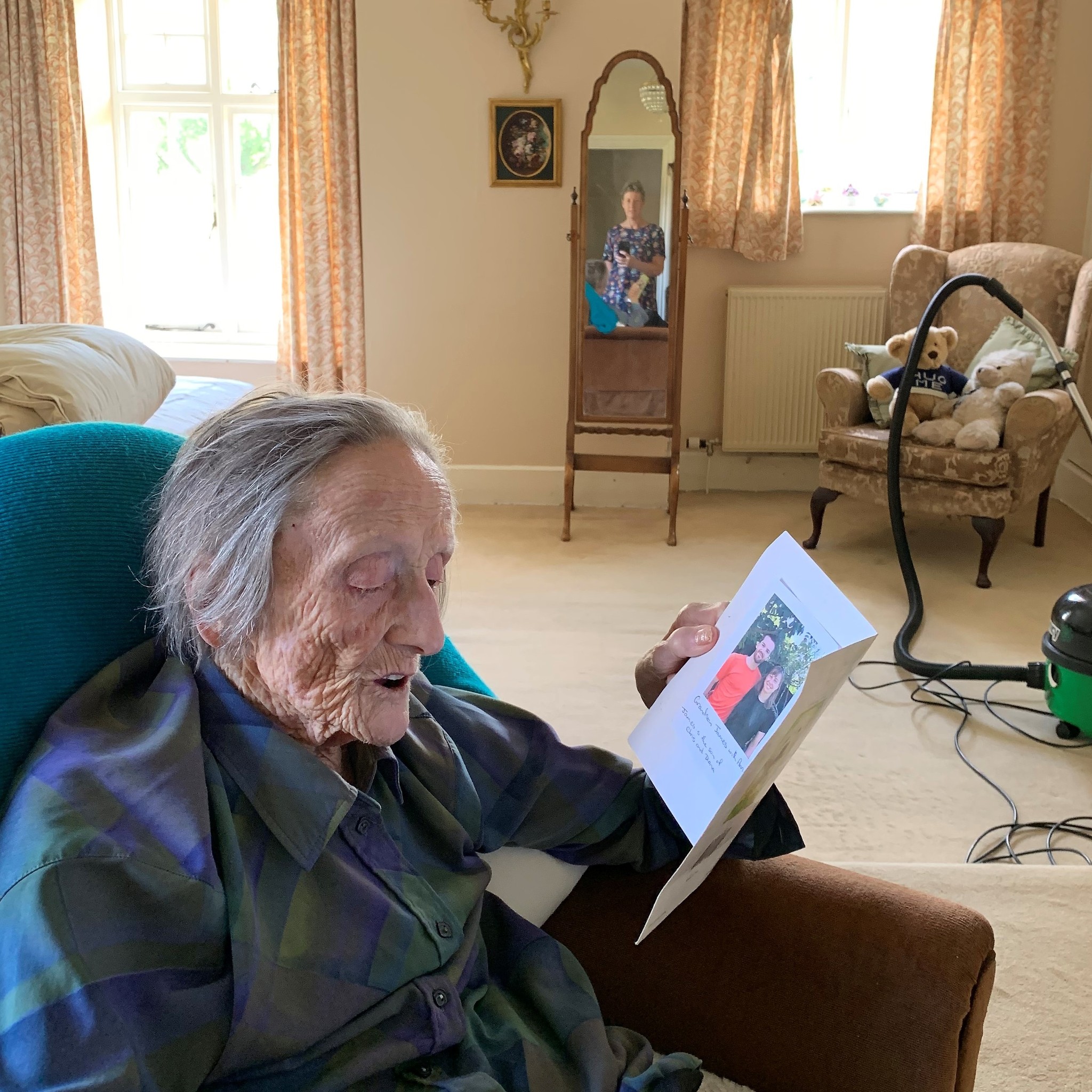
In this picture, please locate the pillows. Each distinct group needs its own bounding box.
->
[843,341,905,427]
[963,315,1078,394]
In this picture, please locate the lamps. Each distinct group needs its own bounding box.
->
[471,0,557,93]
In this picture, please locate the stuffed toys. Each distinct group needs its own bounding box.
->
[911,348,1036,450]
[866,325,972,438]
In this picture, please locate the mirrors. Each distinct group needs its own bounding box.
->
[563,49,689,545]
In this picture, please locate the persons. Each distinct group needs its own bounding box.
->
[0,390,808,1092]
[601,179,666,327]
[705,665,784,759]
[586,259,649,329]
[703,633,779,723]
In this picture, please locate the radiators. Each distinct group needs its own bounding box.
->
[688,282,888,455]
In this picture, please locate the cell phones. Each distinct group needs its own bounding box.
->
[619,241,630,258]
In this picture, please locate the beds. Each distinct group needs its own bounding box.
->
[143,376,255,439]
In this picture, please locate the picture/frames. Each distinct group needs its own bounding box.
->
[489,98,562,188]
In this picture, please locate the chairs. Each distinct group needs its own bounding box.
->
[801,242,1092,590]
[0,420,1001,1091]
